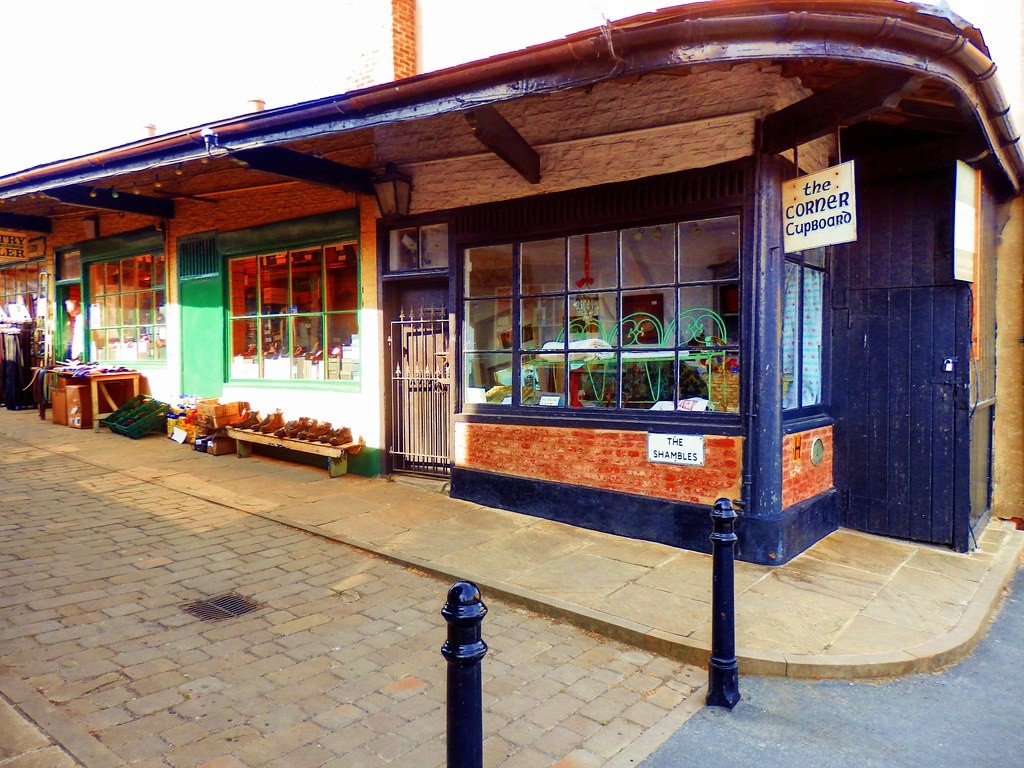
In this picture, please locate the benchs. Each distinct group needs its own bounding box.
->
[224,425,360,478]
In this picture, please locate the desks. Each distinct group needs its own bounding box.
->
[30,367,140,433]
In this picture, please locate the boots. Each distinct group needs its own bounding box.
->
[229,409,354,446]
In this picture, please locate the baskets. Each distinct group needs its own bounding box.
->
[115,400,171,439]
[102,394,154,433]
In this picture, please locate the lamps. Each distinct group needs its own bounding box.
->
[371,164,411,218]
[83,217,98,238]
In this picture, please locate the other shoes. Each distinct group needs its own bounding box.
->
[238,341,344,361]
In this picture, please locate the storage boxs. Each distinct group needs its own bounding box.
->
[50,377,107,430]
[101,395,240,455]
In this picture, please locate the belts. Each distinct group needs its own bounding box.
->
[22,363,59,418]
